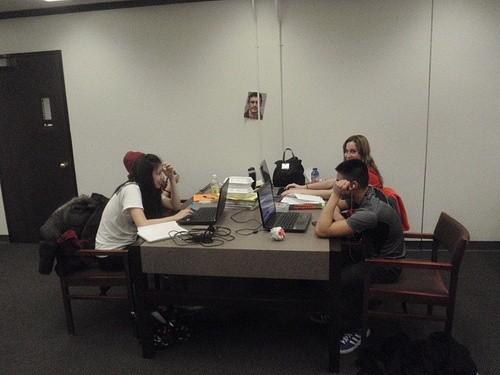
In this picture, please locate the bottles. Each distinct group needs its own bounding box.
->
[211,174,220,195]
[311,168,320,183]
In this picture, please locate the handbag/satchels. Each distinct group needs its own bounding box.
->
[273,148,305,187]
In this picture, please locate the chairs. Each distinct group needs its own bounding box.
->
[40,194,145,341]
[354,214,471,370]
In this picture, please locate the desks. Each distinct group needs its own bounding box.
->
[140,196,343,374]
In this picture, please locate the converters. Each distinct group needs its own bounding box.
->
[193,235,213,243]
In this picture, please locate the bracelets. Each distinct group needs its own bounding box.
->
[306,184,308,189]
[164,190,171,194]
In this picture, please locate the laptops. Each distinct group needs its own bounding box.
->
[260,160,289,197]
[257,179,312,232]
[179,178,229,224]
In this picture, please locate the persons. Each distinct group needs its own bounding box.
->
[244,92,263,120]
[95,153,195,349]
[307,158,406,353]
[123,151,181,216]
[280,134,383,198]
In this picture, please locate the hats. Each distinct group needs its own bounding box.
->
[123,151,144,174]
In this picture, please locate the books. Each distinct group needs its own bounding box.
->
[194,194,219,203]
[289,202,322,210]
[221,193,258,210]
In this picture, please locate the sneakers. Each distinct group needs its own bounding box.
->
[308,311,334,324]
[338,326,370,354]
